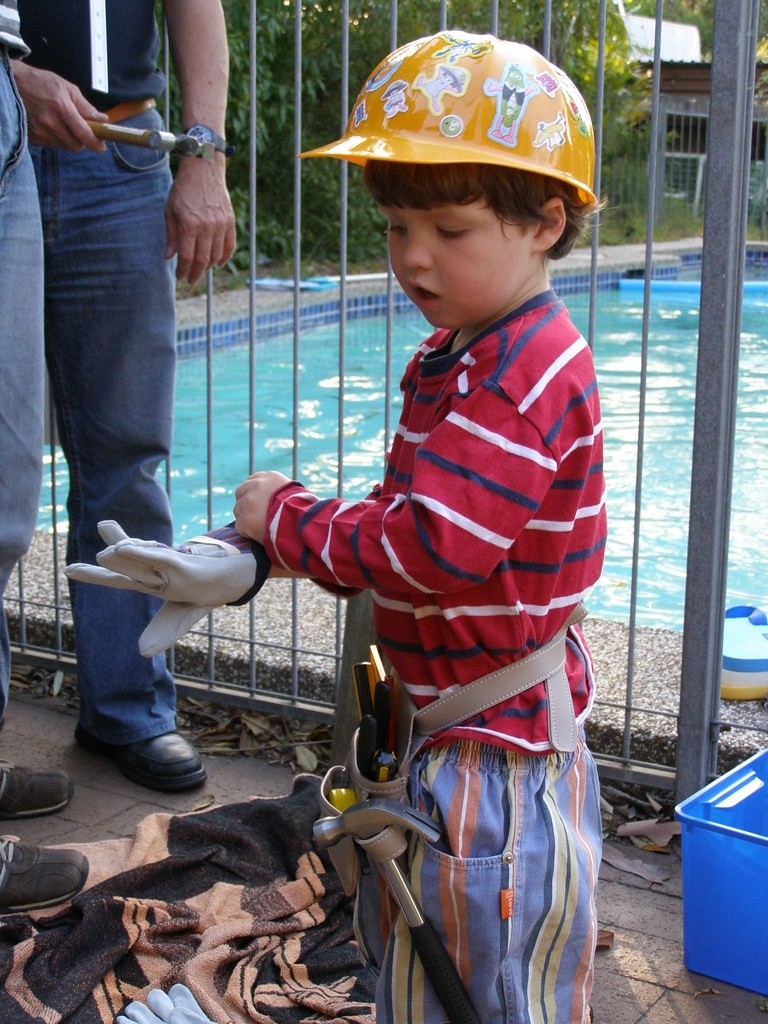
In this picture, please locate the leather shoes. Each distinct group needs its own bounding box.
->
[75,723,208,791]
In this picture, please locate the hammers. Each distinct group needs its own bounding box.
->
[311,798,486,1024]
[82,118,213,161]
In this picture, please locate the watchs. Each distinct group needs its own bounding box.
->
[177,122,236,157]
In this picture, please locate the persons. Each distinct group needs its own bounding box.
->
[0,1,242,793]
[0,47,94,910]
[64,30,611,1024]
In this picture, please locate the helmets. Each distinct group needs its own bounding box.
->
[297,28,597,213]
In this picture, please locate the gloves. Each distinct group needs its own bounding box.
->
[62,520,274,658]
[116,984,216,1024]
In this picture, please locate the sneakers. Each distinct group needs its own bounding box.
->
[0,836,90,913]
[0,757,75,820]
